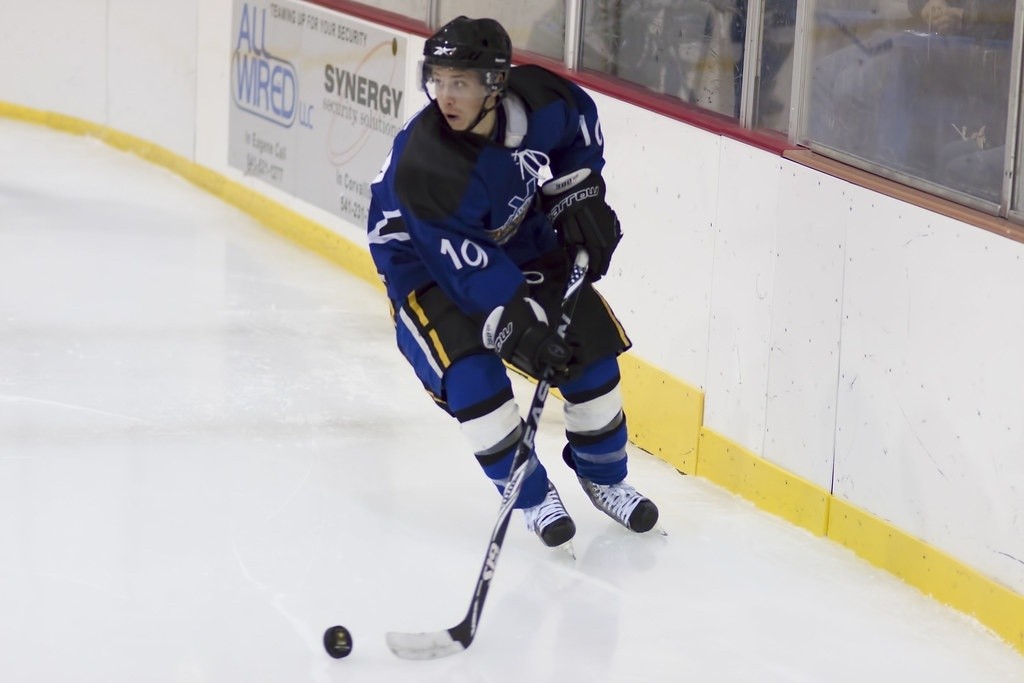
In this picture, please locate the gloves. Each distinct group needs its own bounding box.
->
[542,173,620,284]
[481,296,571,388]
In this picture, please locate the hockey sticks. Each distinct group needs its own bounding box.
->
[380,245,594,660]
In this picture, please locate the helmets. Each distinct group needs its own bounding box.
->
[423,16,510,94]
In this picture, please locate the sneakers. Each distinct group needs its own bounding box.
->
[523,478,575,561]
[561,442,667,536]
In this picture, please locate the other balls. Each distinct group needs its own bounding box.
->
[324,625,352,659]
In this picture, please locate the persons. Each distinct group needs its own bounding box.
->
[664,0,1024,190]
[368,16,667,561]
